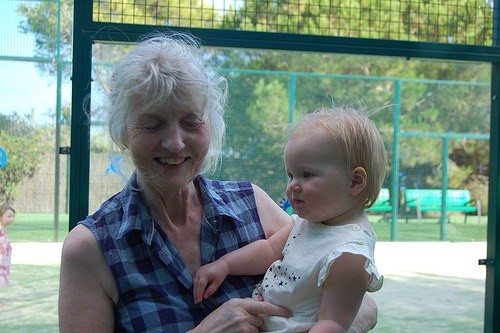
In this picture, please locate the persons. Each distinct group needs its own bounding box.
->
[193,109,386,333]
[0,205,14,308]
[58,38,378,333]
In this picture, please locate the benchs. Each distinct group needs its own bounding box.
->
[365,188,393,223]
[404,188,482,226]
